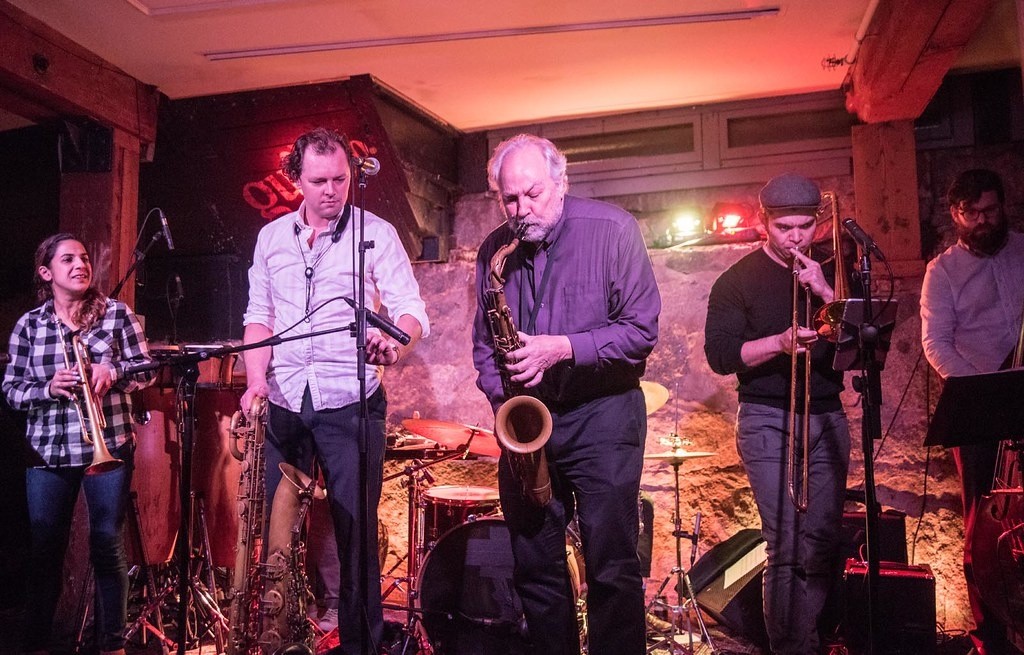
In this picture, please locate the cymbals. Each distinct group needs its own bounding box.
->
[639,381,669,417]
[642,450,719,462]
[400,418,502,457]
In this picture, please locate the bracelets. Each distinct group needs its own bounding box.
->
[383,342,401,367]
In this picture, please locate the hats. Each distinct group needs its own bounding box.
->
[759,174,820,210]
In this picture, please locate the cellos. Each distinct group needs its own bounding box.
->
[964,322,1024,655]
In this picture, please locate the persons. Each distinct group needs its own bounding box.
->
[472,134,661,655]
[703,172,865,655]
[919,168,1024,655]
[2,231,162,655]
[239,127,431,655]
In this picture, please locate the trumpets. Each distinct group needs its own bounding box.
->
[51,308,126,475]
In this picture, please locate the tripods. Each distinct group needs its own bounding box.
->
[645,463,717,655]
[122,387,231,655]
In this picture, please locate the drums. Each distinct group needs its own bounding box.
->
[411,511,585,655]
[419,483,501,563]
[147,341,239,389]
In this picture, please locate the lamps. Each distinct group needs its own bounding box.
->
[711,202,749,237]
[667,203,705,239]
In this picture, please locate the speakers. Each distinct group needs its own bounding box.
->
[136,253,245,345]
[693,541,768,644]
[834,511,936,655]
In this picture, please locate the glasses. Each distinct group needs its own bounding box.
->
[956,202,1002,222]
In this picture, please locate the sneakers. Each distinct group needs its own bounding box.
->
[307,605,318,620]
[319,608,339,631]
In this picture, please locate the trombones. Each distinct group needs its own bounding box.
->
[787,193,854,513]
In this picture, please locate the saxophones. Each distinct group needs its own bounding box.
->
[226,397,326,655]
[482,220,556,508]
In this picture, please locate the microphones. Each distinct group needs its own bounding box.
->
[159,210,174,250]
[351,157,380,176]
[841,217,884,262]
[174,273,184,298]
[468,618,516,628]
[690,512,701,564]
[345,297,412,346]
